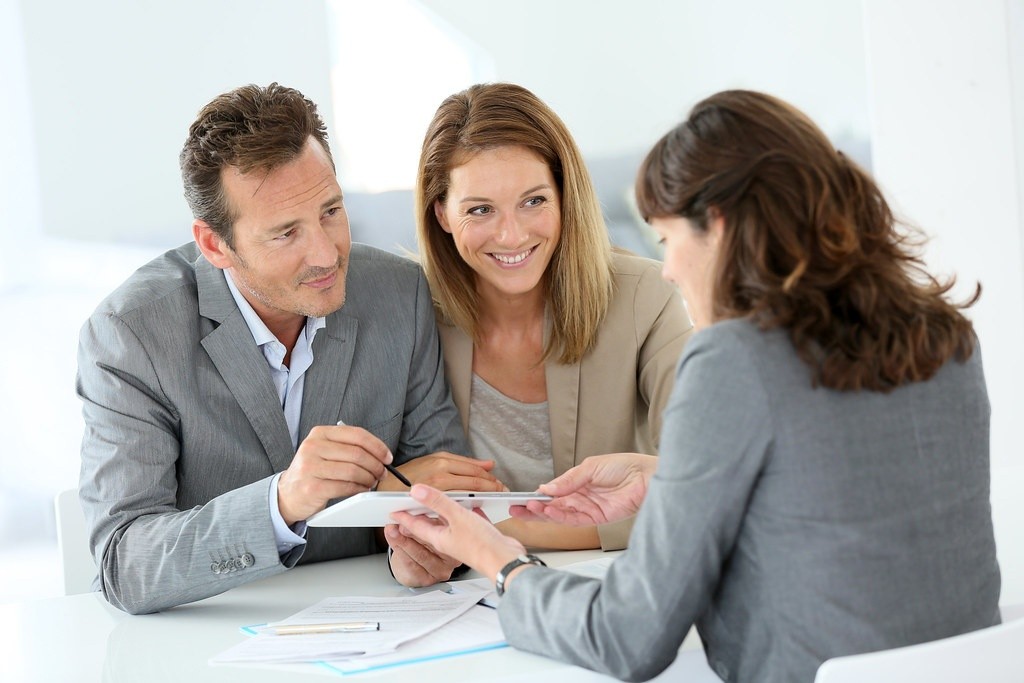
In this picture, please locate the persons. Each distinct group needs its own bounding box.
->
[73,82,479,619]
[375,81,697,552]
[387,84,1004,683]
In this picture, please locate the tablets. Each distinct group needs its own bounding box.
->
[305,491,553,527]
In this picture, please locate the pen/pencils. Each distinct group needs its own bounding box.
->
[259,621,380,635]
[337,420,413,489]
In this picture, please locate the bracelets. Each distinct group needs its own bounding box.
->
[493,553,548,598]
